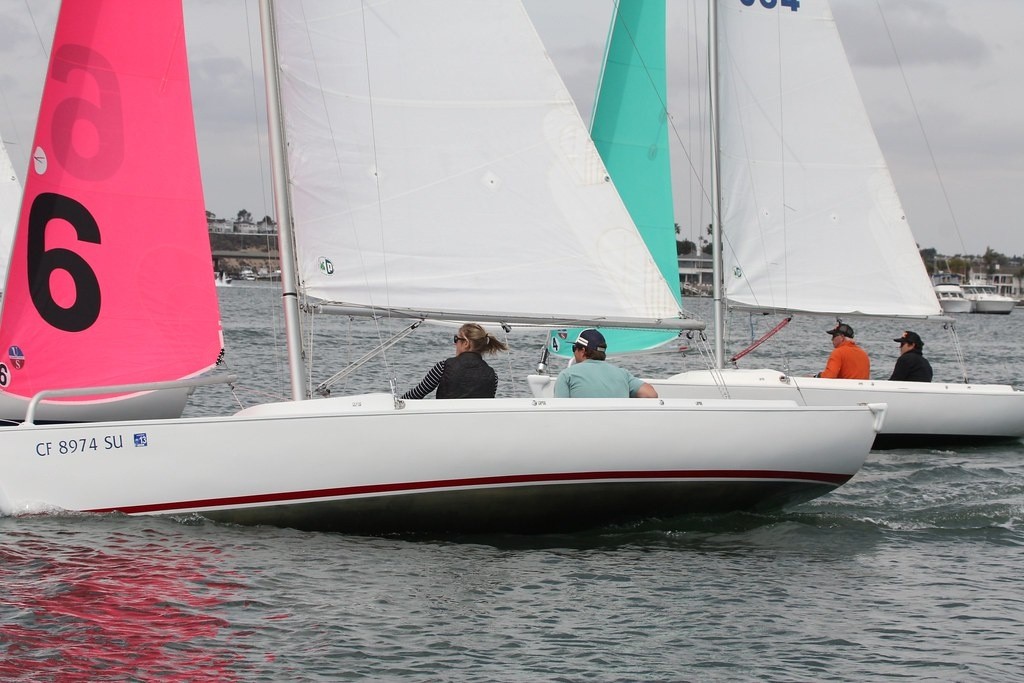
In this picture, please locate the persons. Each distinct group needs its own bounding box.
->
[400,324,508,399]
[803,324,870,379]
[890,331,933,382]
[554,328,658,398]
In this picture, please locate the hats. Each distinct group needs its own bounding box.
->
[894,331,922,346]
[566,329,607,352]
[827,324,854,339]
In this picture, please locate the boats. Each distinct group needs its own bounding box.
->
[930,255,976,313]
[958,273,1021,314]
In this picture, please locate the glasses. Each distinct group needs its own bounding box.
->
[572,344,581,352]
[454,335,466,343]
[832,334,839,338]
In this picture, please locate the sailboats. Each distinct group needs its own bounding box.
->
[0,2,1024,530]
